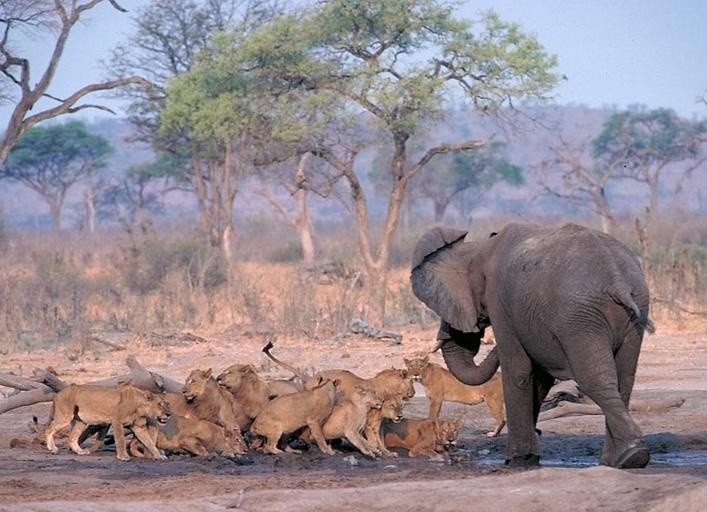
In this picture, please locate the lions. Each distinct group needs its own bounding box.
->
[32,342,506,464]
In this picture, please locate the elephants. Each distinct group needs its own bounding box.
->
[410,220,657,469]
